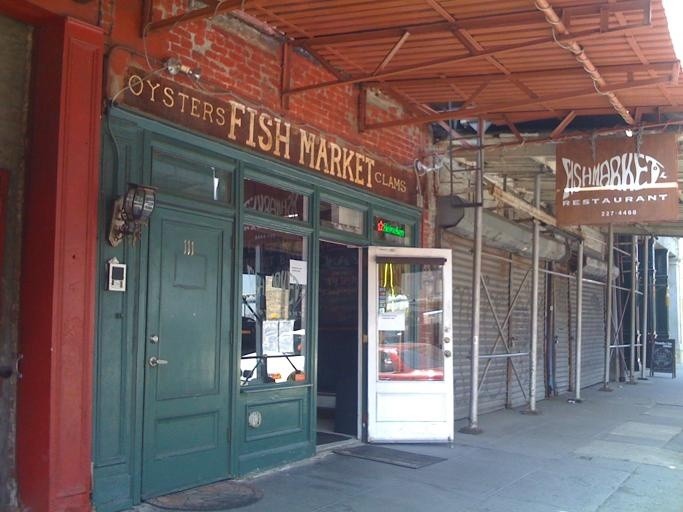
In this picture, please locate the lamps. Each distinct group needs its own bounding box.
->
[167,58,201,81]
[107,184,157,247]
